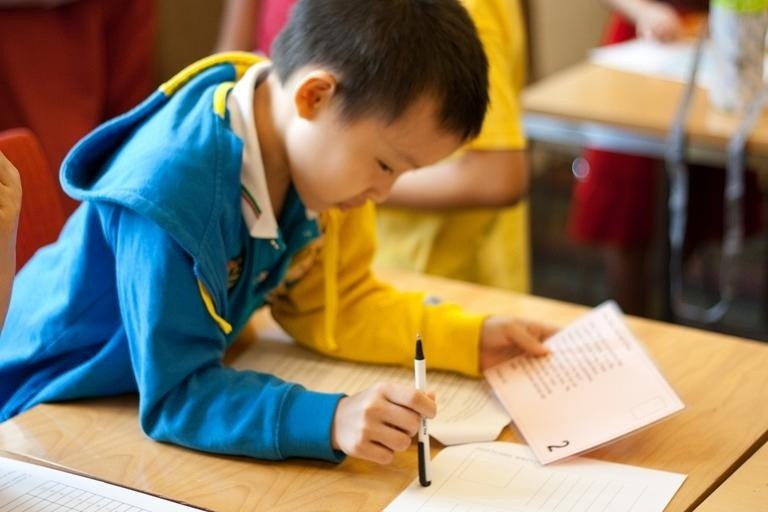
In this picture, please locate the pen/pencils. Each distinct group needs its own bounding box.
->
[414,331,432,486]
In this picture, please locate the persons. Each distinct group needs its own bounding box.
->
[0,149,23,340]
[0,0,166,276]
[566,1,766,321]
[361,0,537,294]
[0,0,563,467]
[210,0,303,54]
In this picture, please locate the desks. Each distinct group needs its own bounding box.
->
[0,263,768,512]
[521,38,767,343]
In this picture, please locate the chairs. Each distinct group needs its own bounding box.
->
[0,128,64,333]
